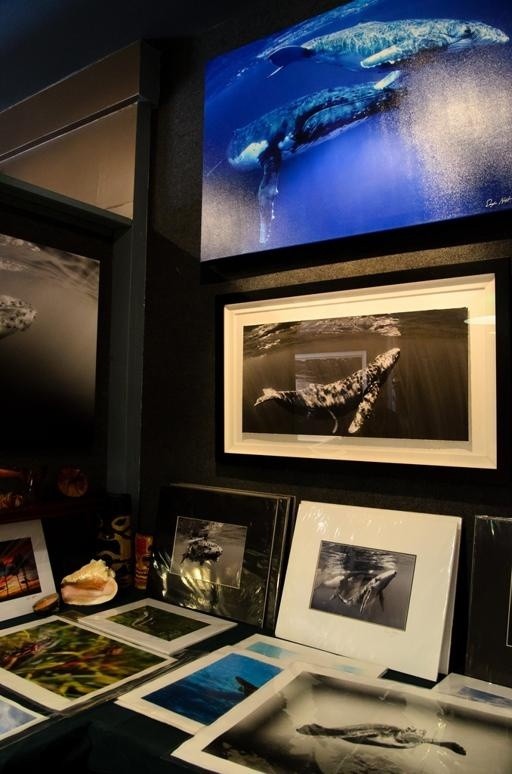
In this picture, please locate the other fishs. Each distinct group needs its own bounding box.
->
[178,539,224,567]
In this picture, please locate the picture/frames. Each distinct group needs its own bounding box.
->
[273,502,467,684]
[0,513,66,627]
[147,483,290,628]
[79,595,239,657]
[215,274,505,478]
